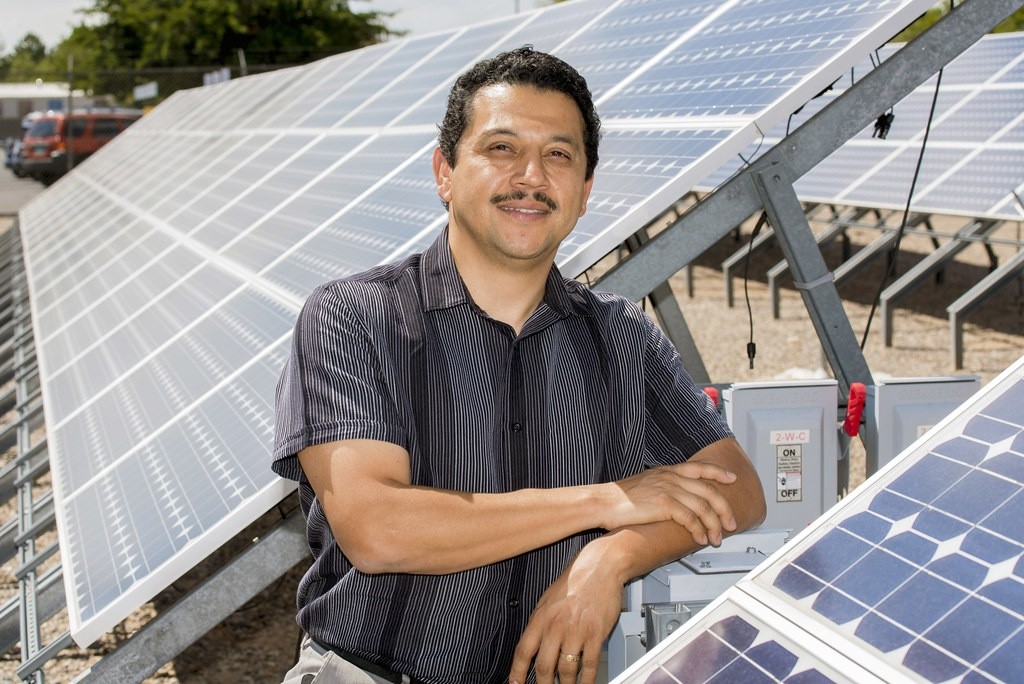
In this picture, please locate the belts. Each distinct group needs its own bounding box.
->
[311,635,425,684]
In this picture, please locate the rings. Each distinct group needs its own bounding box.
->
[560,653,581,662]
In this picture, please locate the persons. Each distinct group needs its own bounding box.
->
[270,45,768,684]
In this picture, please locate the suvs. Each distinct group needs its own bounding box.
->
[20,111,143,185]
